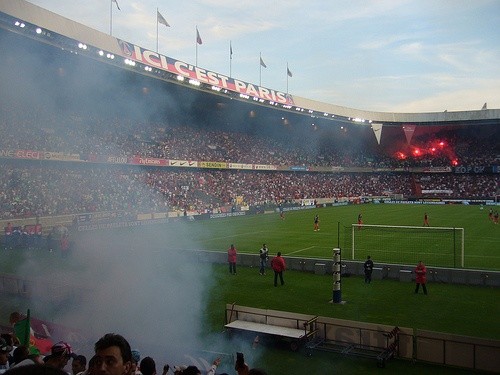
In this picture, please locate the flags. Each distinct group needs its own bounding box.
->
[287,68,292,77]
[196,30,203,44]
[158,11,170,27]
[112,0,120,10]
[260,57,267,68]
[230,46,232,58]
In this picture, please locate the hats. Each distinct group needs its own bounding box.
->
[50,342,72,356]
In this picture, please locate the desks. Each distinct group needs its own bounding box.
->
[223,319,310,352]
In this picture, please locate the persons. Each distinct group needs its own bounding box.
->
[488,208,493,221]
[363,256,373,284]
[479,201,483,213]
[271,251,286,288]
[0,103,500,220]
[4,223,70,260]
[228,243,237,274]
[0,332,267,375]
[313,213,320,232]
[492,210,498,224]
[259,244,269,276]
[357,213,363,230]
[422,211,429,227]
[412,260,428,295]
